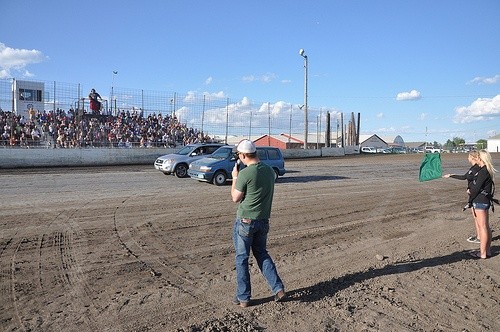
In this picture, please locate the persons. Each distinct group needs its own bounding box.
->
[469,149,499,258]
[231,139,287,307]
[442,150,481,243]
[0,106,222,150]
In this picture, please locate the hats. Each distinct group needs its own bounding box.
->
[236,139,256,153]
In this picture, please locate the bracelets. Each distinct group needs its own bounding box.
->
[233,177,237,179]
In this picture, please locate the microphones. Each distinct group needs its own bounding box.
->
[237,159,240,171]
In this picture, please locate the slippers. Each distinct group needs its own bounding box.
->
[487,255,491,258]
[469,252,486,259]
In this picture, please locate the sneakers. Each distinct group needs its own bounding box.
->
[274,291,286,301]
[232,297,250,307]
[467,236,480,243]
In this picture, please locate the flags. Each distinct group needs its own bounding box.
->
[419,153,442,182]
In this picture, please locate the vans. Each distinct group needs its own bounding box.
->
[153,143,225,179]
[186,145,285,186]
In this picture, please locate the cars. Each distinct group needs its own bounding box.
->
[361,146,470,153]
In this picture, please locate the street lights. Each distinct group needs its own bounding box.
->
[299,48,307,150]
